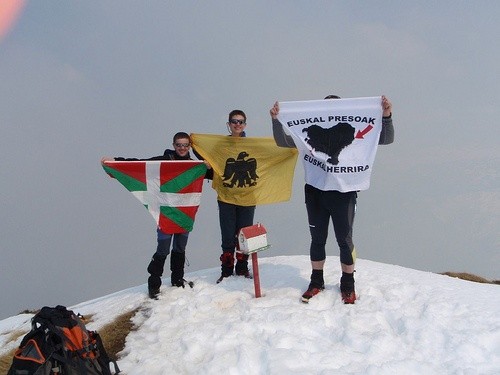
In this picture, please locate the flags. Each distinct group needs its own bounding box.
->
[190,133,299,206]
[275,96,385,193]
[101,160,207,234]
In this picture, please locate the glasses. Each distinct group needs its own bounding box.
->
[229,119,245,124]
[173,143,190,148]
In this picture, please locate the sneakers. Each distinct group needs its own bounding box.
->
[148,288,161,300]
[172,279,193,288]
[340,285,361,304]
[303,282,325,302]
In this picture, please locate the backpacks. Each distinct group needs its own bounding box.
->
[8,305,120,375]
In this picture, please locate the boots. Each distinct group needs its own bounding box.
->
[217,251,234,283]
[236,252,251,278]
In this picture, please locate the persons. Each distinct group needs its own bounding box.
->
[270,94,395,305]
[101,132,214,300]
[189,110,257,284]
